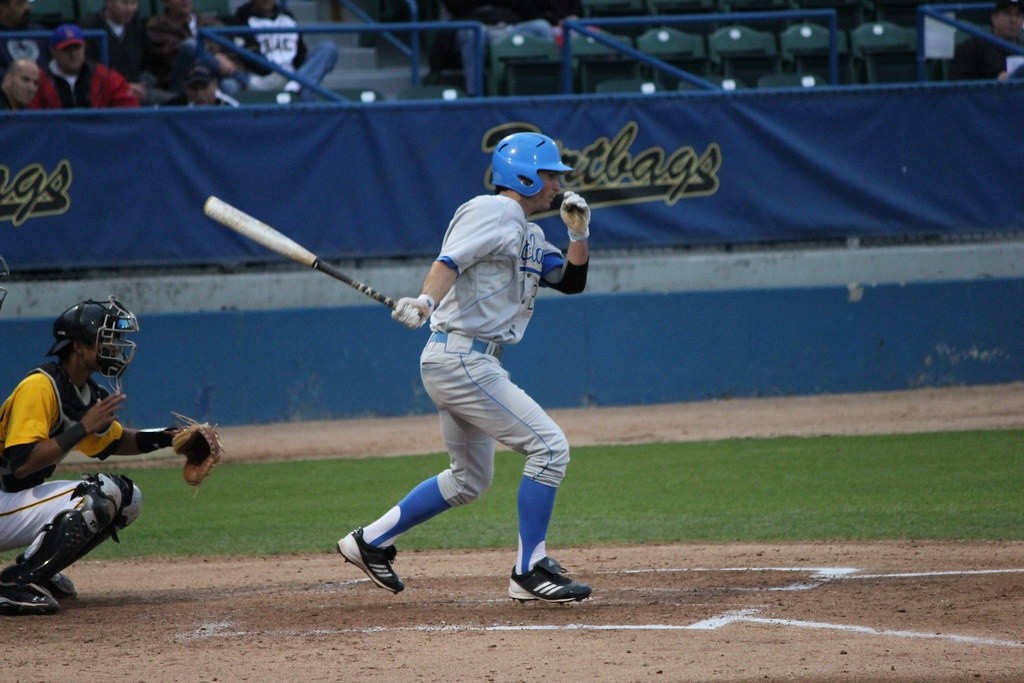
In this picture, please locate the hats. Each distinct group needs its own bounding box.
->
[179,52,219,79]
[50,24,85,50]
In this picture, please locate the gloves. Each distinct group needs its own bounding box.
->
[558,190,592,242]
[390,294,435,330]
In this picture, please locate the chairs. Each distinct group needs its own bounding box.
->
[26,0,1024,114]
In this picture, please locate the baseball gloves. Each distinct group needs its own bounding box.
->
[173,421,219,487]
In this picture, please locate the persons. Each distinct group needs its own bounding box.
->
[441,0,555,97]
[0,297,224,616]
[334,132,592,604]
[937,0,1024,80]
[0,0,338,112]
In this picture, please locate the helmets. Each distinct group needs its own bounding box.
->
[491,132,575,197]
[45,295,110,355]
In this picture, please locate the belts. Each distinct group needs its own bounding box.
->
[430,332,502,359]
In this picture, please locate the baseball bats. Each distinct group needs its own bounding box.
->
[204,194,424,320]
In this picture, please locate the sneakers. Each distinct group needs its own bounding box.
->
[508,556,592,606]
[337,527,405,594]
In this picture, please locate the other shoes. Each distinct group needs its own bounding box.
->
[0,571,60,615]
[36,568,75,598]
